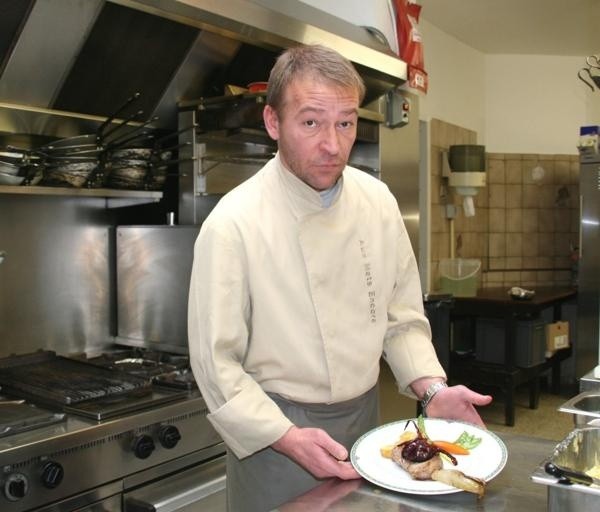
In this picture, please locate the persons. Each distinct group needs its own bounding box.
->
[185,45,493,511]
[272,478,364,510]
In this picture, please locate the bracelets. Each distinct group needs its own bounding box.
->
[418,379,449,409]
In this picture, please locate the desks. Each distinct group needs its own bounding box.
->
[425,285,577,427]
[270,433,563,512]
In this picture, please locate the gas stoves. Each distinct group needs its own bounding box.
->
[0,347,232,512]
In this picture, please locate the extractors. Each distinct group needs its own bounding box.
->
[0,0,410,135]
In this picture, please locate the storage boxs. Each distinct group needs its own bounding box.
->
[474,316,571,370]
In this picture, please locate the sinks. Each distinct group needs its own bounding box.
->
[530,427,599,491]
[560,389,600,416]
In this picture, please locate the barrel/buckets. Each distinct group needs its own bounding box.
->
[442,258,481,298]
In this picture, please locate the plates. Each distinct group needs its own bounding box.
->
[0,150,44,186]
[350,417,508,496]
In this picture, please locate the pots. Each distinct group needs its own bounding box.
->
[46,91,198,186]
